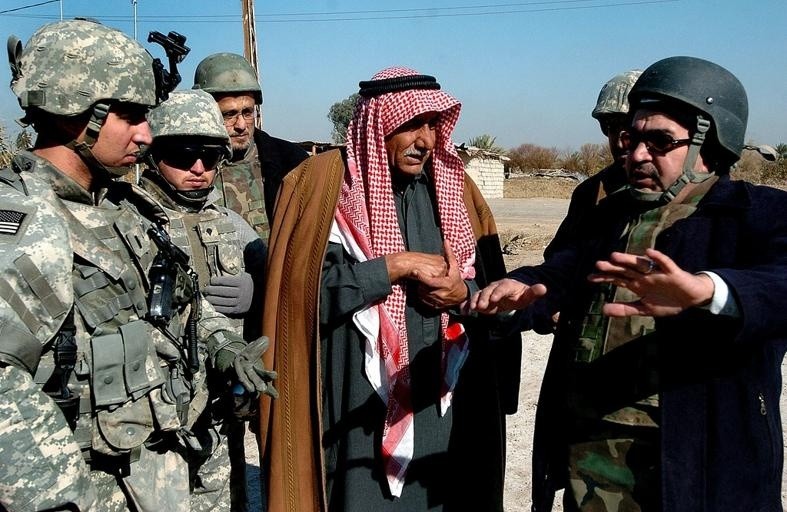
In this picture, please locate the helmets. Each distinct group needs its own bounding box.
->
[6,20,158,115]
[591,71,643,116]
[627,56,749,167]
[136,88,233,166]
[194,53,263,103]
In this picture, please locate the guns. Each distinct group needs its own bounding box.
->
[148,222,200,368]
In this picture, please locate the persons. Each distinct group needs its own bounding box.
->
[458,55,786,510]
[1,16,265,511]
[187,52,312,512]
[246,65,523,511]
[528,69,642,510]
[133,87,264,511]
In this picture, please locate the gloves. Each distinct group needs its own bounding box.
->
[203,267,254,317]
[216,335,282,400]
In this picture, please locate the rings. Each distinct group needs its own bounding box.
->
[644,260,660,276]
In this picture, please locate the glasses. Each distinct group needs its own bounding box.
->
[619,128,693,157]
[221,108,262,125]
[157,145,226,169]
